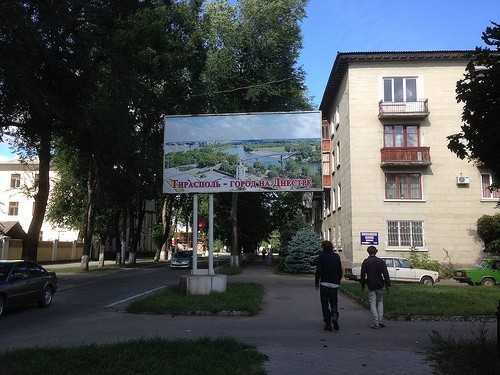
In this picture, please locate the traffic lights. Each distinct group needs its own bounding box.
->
[198,223,203,227]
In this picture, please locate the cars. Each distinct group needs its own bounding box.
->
[0,260,58,316]
[343,256,438,286]
[453,256,500,287]
[170,250,193,270]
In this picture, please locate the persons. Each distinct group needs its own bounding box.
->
[267,252,273,268]
[315,241,342,331]
[361,246,391,330]
[262,248,266,259]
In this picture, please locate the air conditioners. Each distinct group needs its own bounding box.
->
[457,176,469,184]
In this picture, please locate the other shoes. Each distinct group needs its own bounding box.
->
[371,324,379,329]
[379,322,385,326]
[324,325,332,331]
[333,324,339,330]
